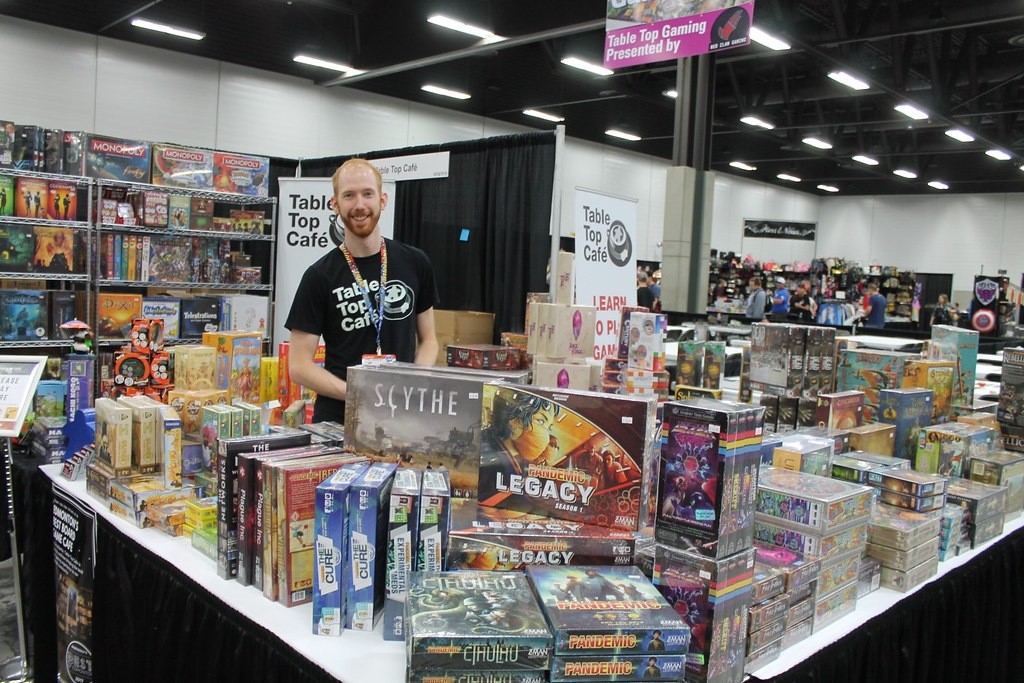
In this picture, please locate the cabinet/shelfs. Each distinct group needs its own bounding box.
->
[0,166,95,349]
[96,178,278,402]
[636,258,915,321]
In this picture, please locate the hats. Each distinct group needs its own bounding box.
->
[775,277,786,285]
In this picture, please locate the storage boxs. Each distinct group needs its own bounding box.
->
[0,294,1024,683]
[0,119,271,286]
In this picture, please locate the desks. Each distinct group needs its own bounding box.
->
[34,465,1024,683]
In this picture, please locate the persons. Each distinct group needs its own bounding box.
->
[644,656,661,678]
[550,569,645,602]
[860,283,886,329]
[637,269,661,314]
[463,588,518,629]
[6,124,15,151]
[284,158,440,426]
[930,293,958,328]
[478,387,628,508]
[960,500,972,524]
[740,387,752,403]
[709,277,818,325]
[647,630,664,651]
[53,231,65,247]
[449,541,524,571]
[0,188,72,220]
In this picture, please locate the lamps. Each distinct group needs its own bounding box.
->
[130,14,207,41]
